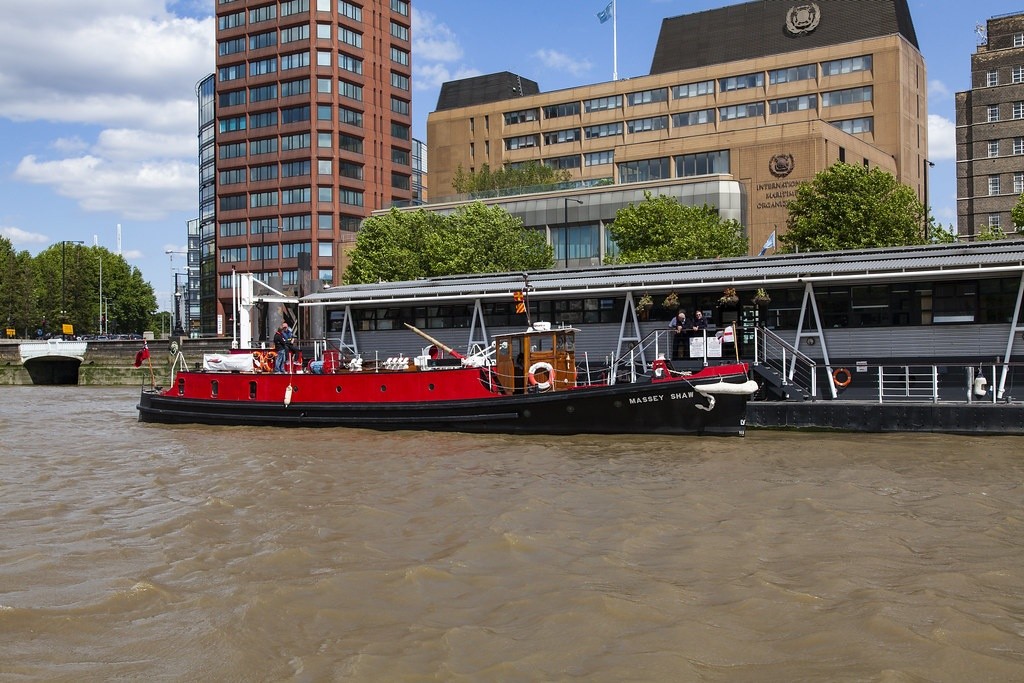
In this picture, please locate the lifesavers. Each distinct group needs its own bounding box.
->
[832,368,852,388]
[528,361,556,390]
[251,351,277,372]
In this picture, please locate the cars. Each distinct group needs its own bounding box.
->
[37,331,143,341]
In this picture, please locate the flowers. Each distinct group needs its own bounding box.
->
[635,285,771,317]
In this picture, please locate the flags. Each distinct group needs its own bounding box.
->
[715,326,734,343]
[134,347,149,368]
[515,303,526,313]
[597,1,613,24]
[514,292,524,301]
[758,231,775,256]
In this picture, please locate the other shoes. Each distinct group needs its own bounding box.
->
[281,371,288,374]
[275,371,282,374]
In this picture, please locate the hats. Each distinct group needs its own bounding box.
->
[282,323,288,328]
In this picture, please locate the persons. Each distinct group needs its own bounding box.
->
[274,327,288,374]
[690,310,707,331]
[282,323,299,364]
[669,313,688,361]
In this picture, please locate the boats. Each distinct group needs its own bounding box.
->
[137,262,757,438]
[297,242,1024,437]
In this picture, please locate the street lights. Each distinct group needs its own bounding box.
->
[924,159,935,240]
[63,241,85,341]
[565,198,584,268]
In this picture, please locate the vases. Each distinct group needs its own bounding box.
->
[644,304,652,310]
[727,300,739,305]
[758,299,770,304]
[668,303,680,309]
